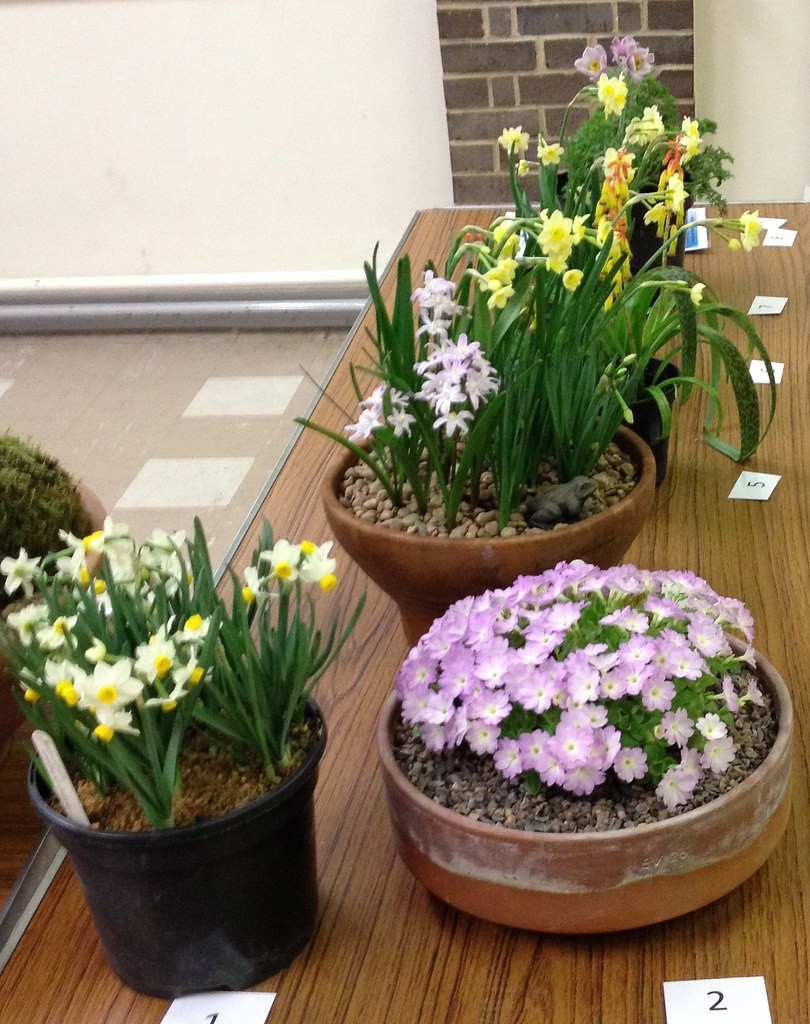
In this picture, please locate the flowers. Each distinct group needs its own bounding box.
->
[1,28,781,812]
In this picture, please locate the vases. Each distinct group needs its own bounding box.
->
[323,413,655,653]
[599,357,681,483]
[373,634,801,932]
[27,696,327,998]
[546,167,695,272]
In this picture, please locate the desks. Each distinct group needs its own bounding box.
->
[2,202,810,1024]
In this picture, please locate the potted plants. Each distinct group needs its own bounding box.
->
[1,427,117,741]
